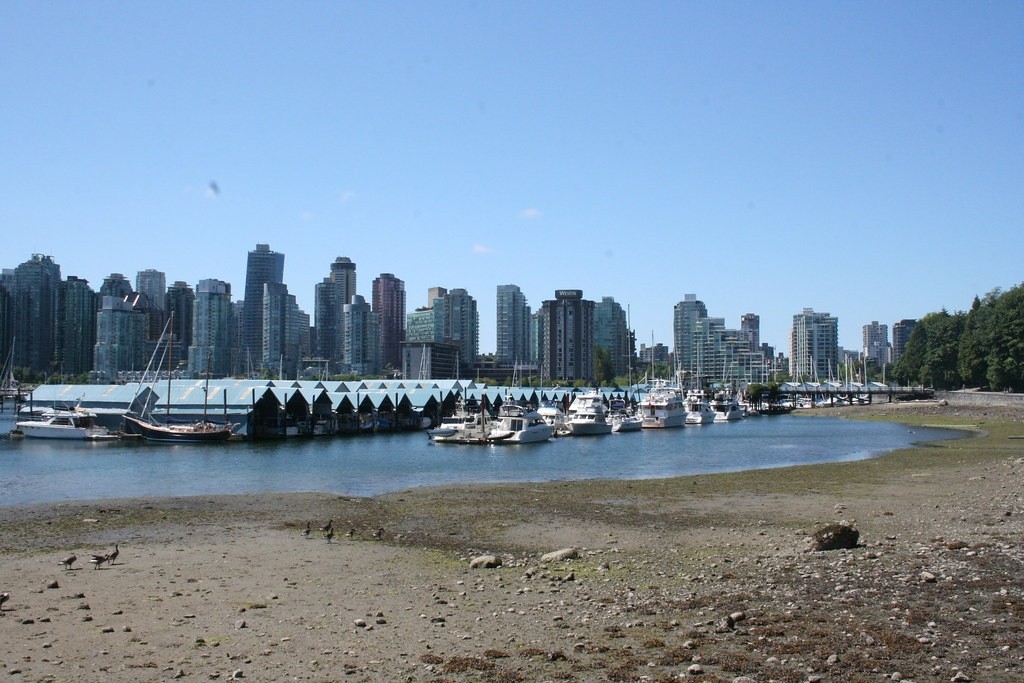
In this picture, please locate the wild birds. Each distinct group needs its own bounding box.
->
[301,519,386,544]
[86,544,120,571]
[57,555,77,571]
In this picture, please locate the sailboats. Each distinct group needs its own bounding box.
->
[0,336,35,396]
[117,310,233,443]
[776,352,887,408]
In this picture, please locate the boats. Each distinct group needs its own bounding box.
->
[637,386,688,428]
[709,354,744,421]
[16,412,111,440]
[533,405,567,434]
[564,390,613,434]
[683,340,716,424]
[427,396,493,444]
[486,403,554,445]
[739,404,748,416]
[553,428,570,435]
[604,305,643,432]
